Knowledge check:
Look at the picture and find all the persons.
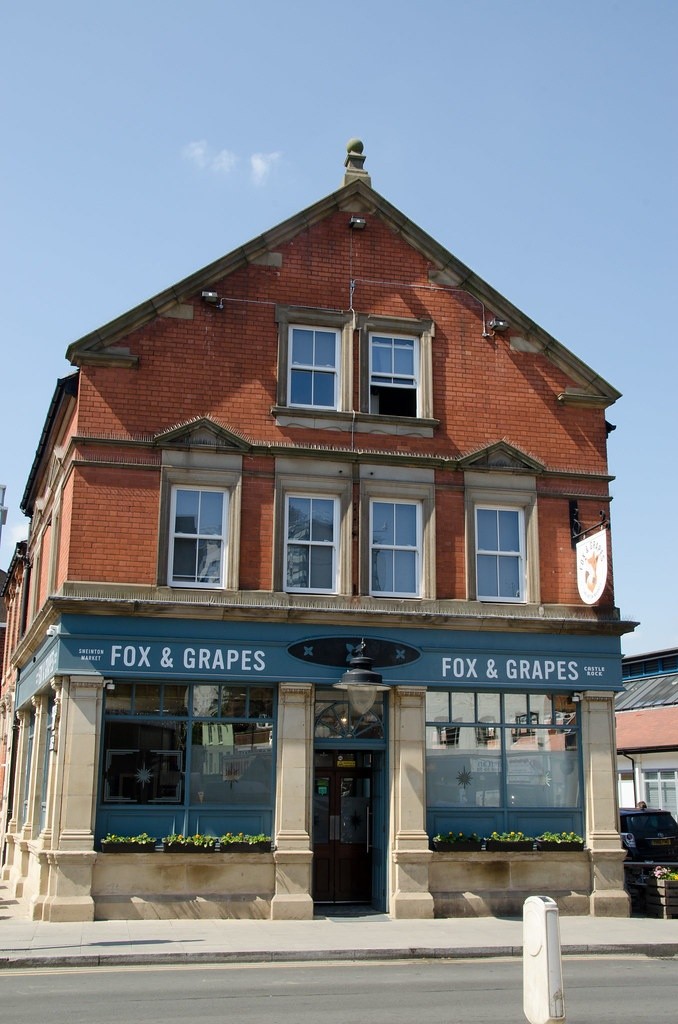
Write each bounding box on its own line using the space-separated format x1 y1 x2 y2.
637 801 647 809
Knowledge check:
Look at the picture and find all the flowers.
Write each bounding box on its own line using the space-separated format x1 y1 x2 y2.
220 832 272 846
162 833 215 847
649 866 677 880
434 831 480 844
100 833 158 844
541 831 584 844
487 831 534 842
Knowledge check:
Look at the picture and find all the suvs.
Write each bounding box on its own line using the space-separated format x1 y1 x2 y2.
618 801 678 896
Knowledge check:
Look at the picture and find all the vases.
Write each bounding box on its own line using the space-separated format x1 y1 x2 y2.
217 838 273 852
97 842 156 852
163 842 217 853
645 877 678 919
486 839 534 850
535 837 585 851
429 838 482 851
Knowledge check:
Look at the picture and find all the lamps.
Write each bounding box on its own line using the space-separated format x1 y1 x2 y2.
489 319 510 332
350 214 367 231
331 641 391 716
200 290 219 304
